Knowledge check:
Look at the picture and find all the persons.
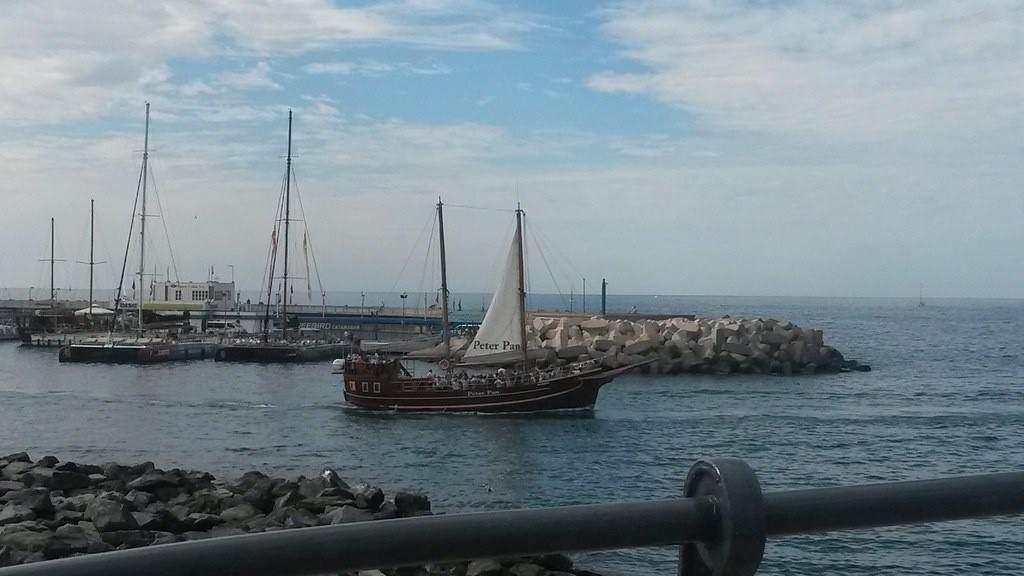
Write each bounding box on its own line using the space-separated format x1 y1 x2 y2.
231 335 341 347
352 349 556 391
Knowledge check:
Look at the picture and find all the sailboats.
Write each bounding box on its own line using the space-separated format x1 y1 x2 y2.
332 194 658 417
20 95 472 364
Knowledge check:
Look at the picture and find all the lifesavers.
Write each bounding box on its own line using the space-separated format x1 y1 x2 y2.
439 359 450 371
222 338 229 346
